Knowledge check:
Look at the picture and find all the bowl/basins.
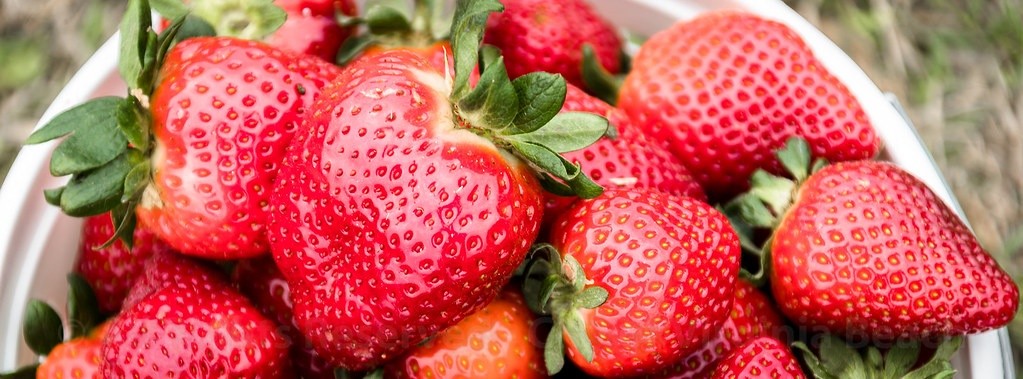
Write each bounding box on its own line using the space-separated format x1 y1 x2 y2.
1 0 1017 379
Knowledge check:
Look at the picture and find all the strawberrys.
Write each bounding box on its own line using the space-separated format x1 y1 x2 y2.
0 0 1021 379
